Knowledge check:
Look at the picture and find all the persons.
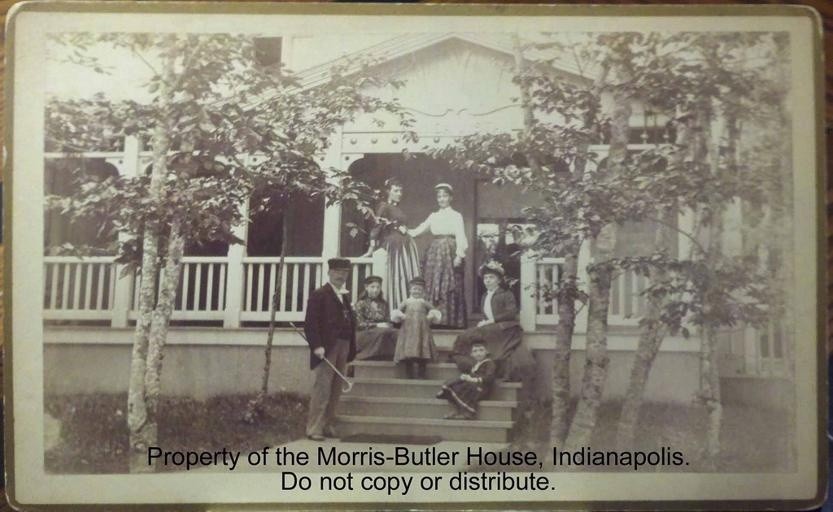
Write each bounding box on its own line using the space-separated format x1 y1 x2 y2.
304 257 356 441
362 178 421 319
353 276 393 363
390 277 443 378
436 339 497 419
451 260 524 381
408 182 469 327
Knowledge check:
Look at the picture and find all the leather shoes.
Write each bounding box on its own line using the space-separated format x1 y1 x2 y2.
309 434 326 440
322 425 340 438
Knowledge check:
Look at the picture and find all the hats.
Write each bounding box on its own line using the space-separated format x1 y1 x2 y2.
365 277 382 282
406 277 426 290
327 259 353 272
470 337 488 348
477 261 506 284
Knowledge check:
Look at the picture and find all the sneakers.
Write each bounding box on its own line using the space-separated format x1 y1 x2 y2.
435 387 451 399
443 407 471 420
